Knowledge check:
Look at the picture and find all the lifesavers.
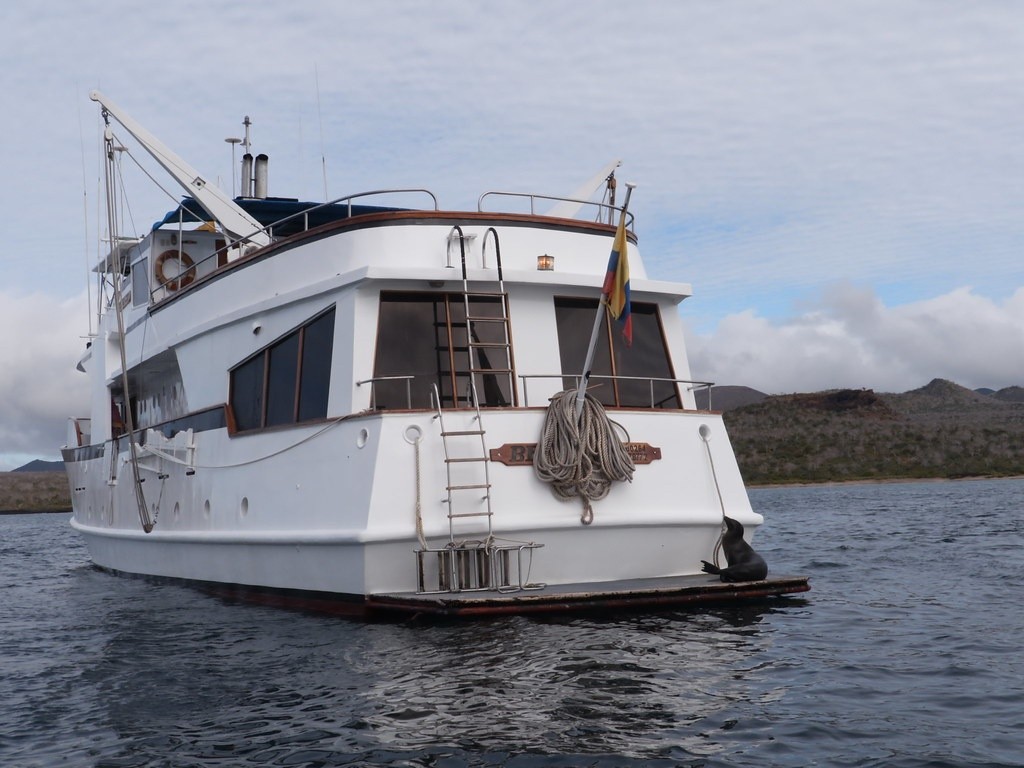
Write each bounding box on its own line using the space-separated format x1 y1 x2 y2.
155 248 196 290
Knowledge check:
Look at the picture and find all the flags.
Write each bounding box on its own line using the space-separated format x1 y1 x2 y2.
600 207 633 351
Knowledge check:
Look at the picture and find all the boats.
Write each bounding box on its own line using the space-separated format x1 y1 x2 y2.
59 91 814 623
